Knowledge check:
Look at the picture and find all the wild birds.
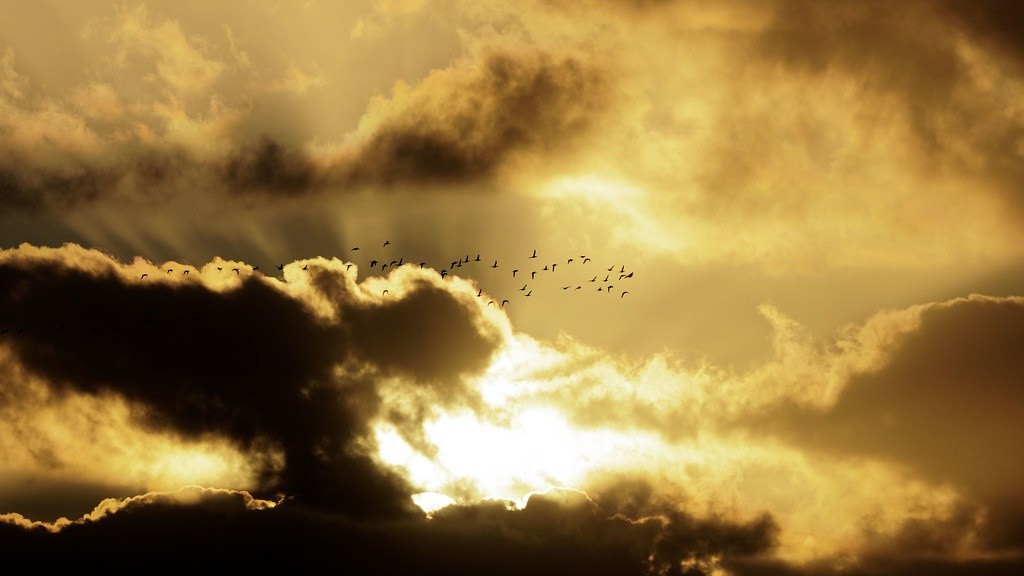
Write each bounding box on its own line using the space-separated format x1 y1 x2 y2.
138 238 637 308
